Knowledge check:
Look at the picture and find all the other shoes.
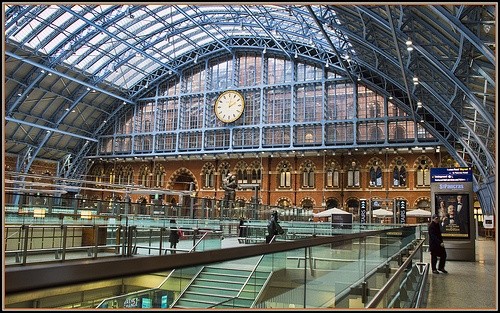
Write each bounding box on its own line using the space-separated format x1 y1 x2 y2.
438 269 448 273
432 270 439 274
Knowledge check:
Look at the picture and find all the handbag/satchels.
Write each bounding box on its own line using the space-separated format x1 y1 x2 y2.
177 230 182 237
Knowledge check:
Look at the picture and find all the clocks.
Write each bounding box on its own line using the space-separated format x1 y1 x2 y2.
213 89 246 123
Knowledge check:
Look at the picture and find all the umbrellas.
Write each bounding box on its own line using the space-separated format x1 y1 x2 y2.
281 209 314 216
314 208 349 218
405 209 431 223
366 209 394 223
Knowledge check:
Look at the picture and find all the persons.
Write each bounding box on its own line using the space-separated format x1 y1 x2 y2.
169 218 179 253
222 173 237 216
309 216 313 222
238 217 244 237
438 195 468 234
428 215 447 274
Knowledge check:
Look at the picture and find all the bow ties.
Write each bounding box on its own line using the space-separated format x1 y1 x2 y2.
458 203 461 205
450 217 454 219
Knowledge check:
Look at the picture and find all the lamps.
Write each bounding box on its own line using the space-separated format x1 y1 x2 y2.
324 58 329 68
193 53 199 64
417 98 422 109
262 44 267 54
294 49 298 58
168 63 174 75
144 77 150 89
405 35 414 53
357 73 362 82
412 72 420 85
389 92 394 100
